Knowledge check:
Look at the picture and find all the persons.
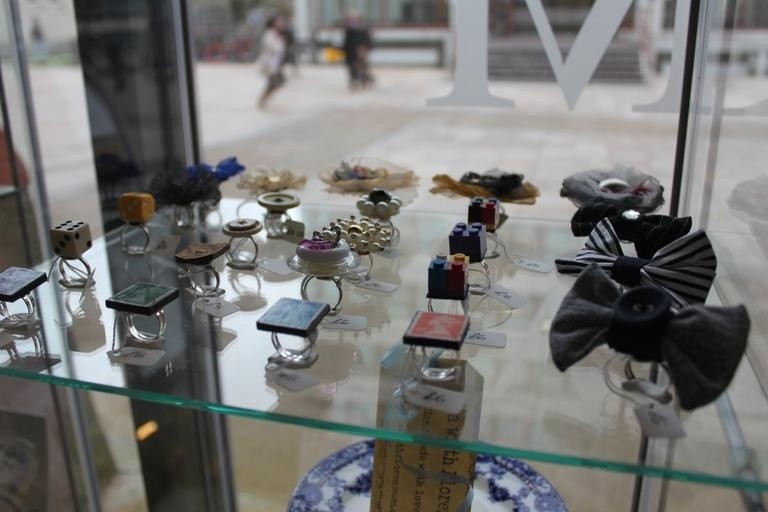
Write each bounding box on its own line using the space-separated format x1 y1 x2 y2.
341 9 375 94
254 12 296 108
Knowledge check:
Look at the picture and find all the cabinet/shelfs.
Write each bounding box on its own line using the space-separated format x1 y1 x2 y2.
0 0 767 512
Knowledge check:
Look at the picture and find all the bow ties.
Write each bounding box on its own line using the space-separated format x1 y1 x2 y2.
548 261 752 413
555 217 718 310
571 203 693 260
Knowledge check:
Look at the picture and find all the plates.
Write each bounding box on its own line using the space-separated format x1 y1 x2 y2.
285 438 567 512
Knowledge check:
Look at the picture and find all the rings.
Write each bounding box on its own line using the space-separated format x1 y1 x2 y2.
1 189 509 413
